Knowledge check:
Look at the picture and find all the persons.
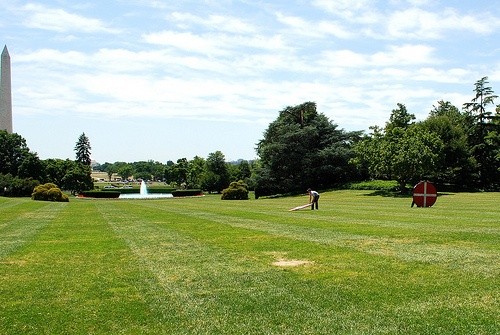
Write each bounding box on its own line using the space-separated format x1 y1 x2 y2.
307 188 320 211
409 187 421 208
